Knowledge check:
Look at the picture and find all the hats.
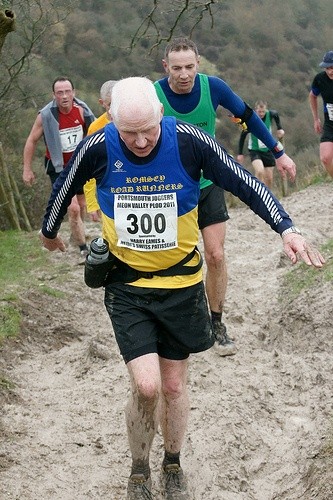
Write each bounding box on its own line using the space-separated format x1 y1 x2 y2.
318 51 333 68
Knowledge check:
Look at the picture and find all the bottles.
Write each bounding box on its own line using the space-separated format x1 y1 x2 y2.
86 237 109 264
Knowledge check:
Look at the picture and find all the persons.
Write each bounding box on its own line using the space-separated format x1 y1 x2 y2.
236 99 285 191
152 38 295 356
308 51 333 181
23 77 121 256
42 76 326 500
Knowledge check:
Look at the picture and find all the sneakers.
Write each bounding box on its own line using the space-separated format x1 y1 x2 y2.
126 471 152 500
211 321 237 356
78 247 88 265
161 463 184 500
